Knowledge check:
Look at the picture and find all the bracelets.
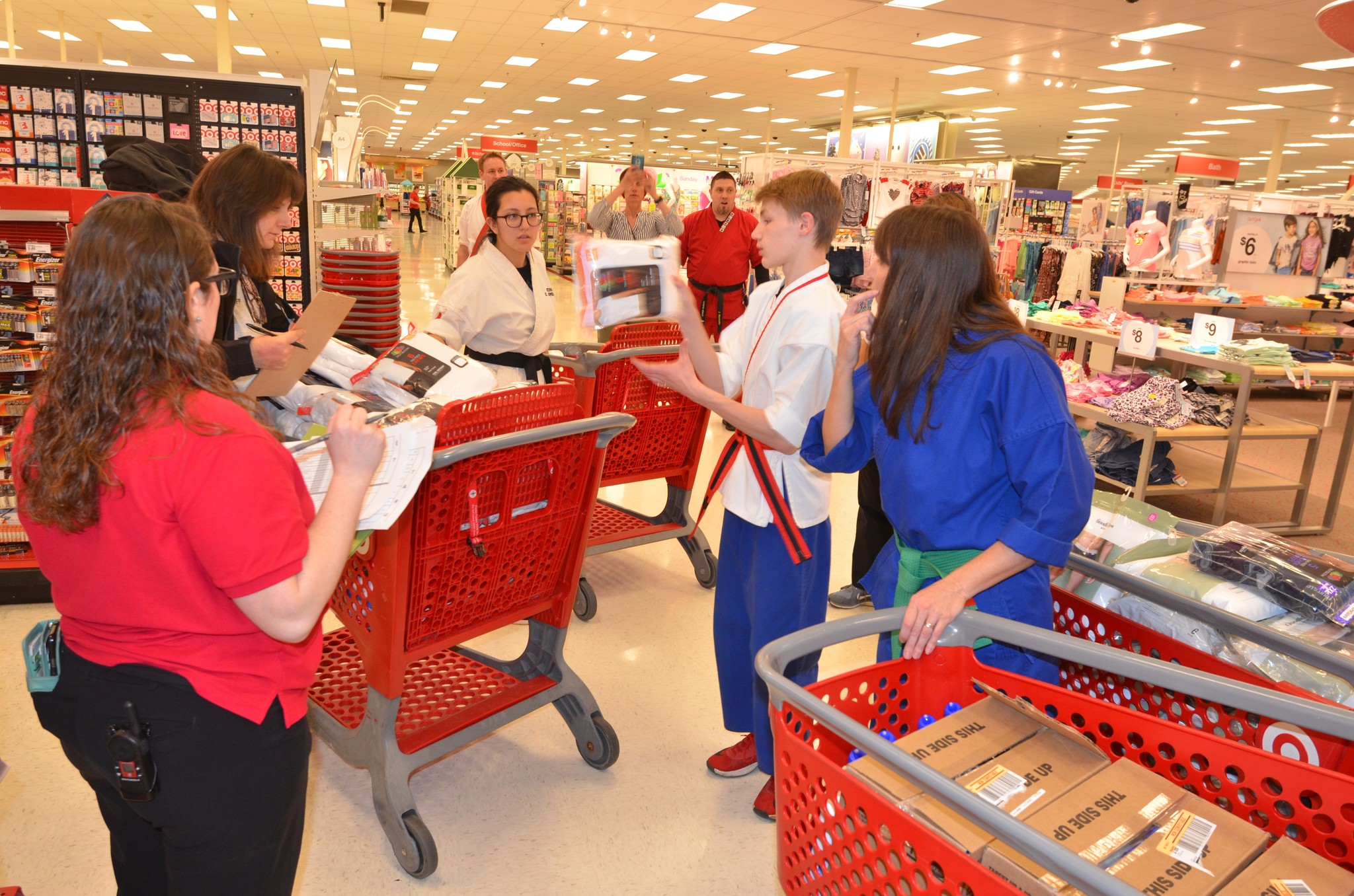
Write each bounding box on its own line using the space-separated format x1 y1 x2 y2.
654 197 663 204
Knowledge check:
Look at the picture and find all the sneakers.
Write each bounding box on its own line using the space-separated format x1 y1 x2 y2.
706 733 759 778
828 585 873 609
753 775 776 821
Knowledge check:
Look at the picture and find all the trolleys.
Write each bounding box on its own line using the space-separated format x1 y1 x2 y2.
752 599 1354 896
1046 517 1354 878
539 318 719 624
297 378 640 880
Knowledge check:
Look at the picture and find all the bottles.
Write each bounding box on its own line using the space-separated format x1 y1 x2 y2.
372 237 378 251
363 237 369 251
354 237 360 251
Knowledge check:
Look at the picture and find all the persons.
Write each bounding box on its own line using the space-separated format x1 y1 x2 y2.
188 144 381 381
423 193 429 211
457 151 541 268
408 185 428 233
663 178 680 216
557 179 564 191
800 205 1096 718
1275 215 1324 276
1170 218 1213 279
631 170 848 822
1123 210 1170 271
12 193 387 896
698 179 712 211
676 170 770 343
828 192 976 609
1088 203 1102 234
423 176 556 384
587 165 684 241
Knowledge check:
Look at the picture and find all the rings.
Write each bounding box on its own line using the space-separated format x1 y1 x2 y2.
924 622 935 628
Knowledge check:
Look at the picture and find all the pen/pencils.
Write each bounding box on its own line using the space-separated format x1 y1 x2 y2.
285 413 390 452
245 322 310 349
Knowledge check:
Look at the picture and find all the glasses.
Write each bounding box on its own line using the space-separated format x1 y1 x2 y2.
498 213 543 227
205 267 237 298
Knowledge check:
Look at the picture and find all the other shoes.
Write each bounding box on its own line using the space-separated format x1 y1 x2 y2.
408 230 415 233
420 229 427 233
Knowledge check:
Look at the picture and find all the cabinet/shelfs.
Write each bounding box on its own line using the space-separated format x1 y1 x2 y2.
1026 278 1354 537
317 176 581 273
